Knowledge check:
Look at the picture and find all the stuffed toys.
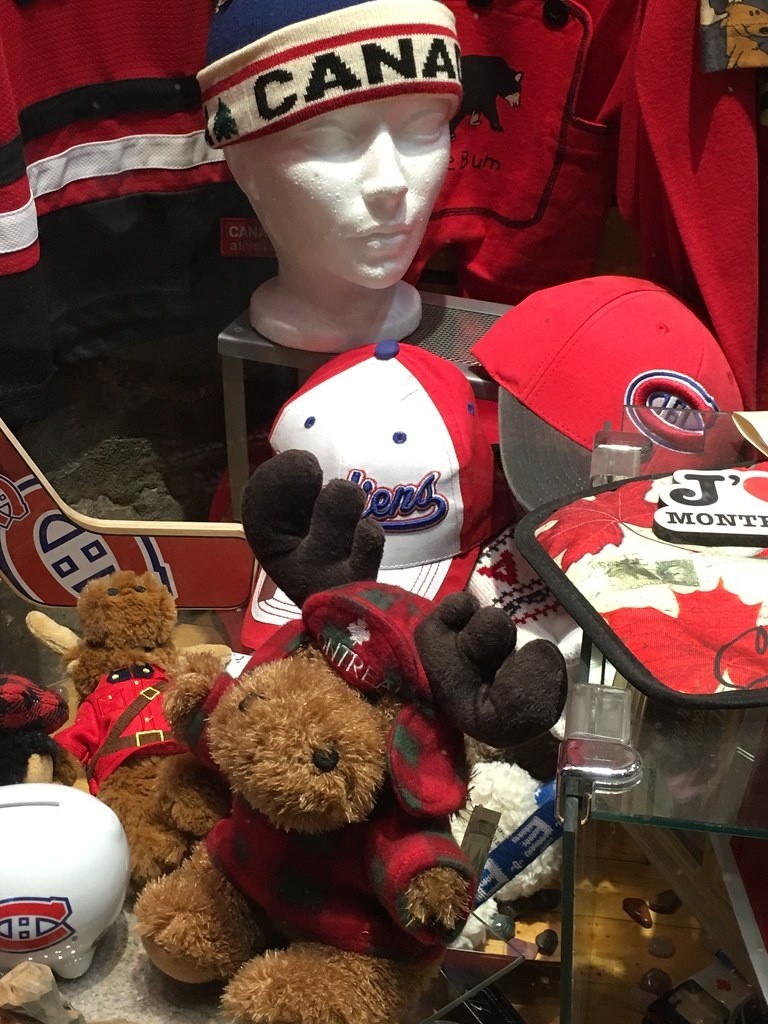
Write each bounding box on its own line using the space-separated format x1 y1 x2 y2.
26 568 222 885
134 450 567 1024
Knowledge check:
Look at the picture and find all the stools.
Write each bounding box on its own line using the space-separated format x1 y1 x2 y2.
215 290 514 521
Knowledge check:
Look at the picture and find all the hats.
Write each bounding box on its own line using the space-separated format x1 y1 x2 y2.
193 1 472 151
235 339 497 656
468 272 748 517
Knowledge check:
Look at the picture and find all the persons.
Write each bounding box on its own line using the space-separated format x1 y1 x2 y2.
201 1 465 354
